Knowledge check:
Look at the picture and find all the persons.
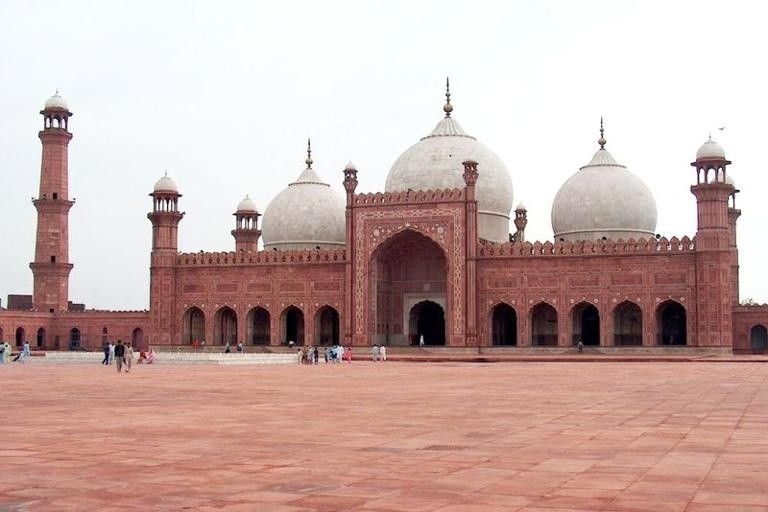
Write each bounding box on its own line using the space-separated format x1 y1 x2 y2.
288 338 295 348
379 345 387 361
0 340 31 366
225 340 231 352
371 344 380 364
419 333 424 348
297 343 353 365
101 340 158 372
578 340 583 352
235 340 246 353
